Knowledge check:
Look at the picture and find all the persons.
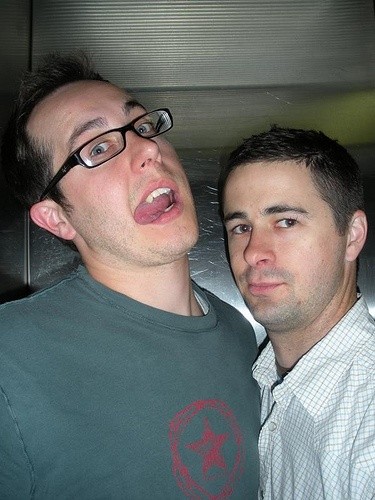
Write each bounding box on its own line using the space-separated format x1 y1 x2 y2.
0 49 267 499
213 123 375 500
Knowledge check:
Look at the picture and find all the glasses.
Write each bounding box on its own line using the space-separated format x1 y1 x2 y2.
38 108 173 203
